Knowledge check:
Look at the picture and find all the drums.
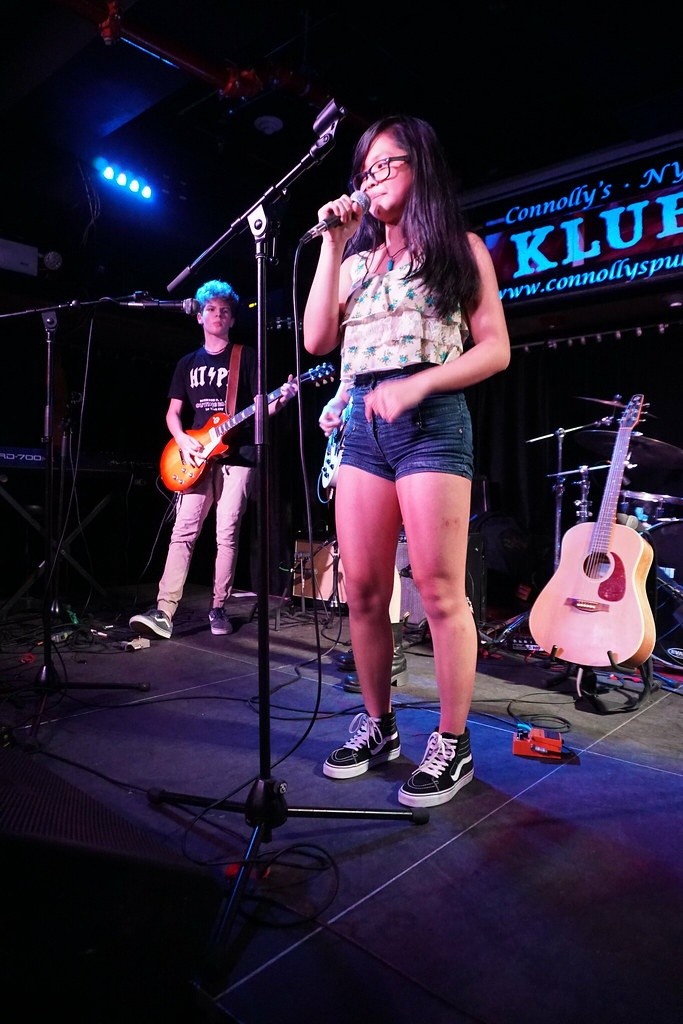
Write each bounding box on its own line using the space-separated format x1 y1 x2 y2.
639 521 683 668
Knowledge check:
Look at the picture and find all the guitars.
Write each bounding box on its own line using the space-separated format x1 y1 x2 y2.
160 363 344 493
529 393 656 671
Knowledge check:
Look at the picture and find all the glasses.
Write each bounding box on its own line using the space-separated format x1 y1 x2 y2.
353 156 410 192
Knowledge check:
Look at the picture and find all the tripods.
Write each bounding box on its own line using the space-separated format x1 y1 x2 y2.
147 98 430 978
295 533 350 628
0 289 150 749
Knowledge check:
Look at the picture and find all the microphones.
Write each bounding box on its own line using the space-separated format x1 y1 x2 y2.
120 297 200 315
300 191 370 245
291 568 318 576
399 570 413 579
607 461 630 486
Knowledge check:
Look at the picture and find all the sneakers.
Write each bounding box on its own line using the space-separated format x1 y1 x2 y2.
209 607 233 635
323 707 400 778
129 609 174 638
398 727 474 807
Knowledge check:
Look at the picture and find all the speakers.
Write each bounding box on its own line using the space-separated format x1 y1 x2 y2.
291 532 350 614
387 533 485 631
0 745 230 1024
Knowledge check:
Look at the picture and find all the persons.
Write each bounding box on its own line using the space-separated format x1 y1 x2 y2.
315 287 409 695
127 278 304 639
300 117 512 807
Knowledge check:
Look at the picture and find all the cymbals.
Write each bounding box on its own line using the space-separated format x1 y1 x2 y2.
579 397 656 420
575 430 681 464
